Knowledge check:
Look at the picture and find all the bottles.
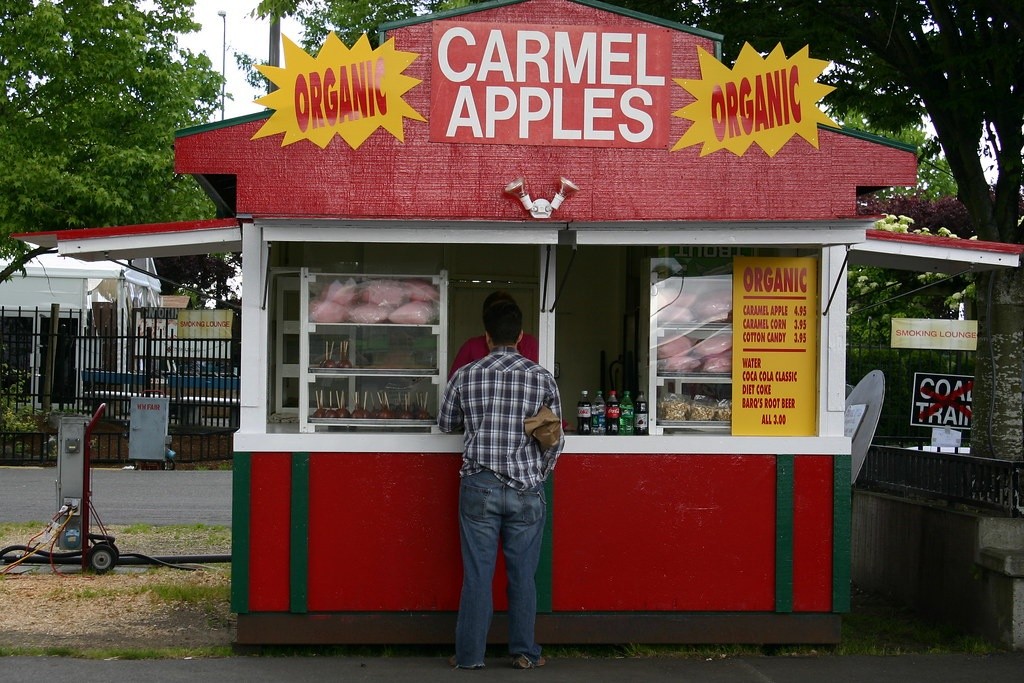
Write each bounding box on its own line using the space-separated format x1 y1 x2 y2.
619 390 634 434
592 390 606 434
606 390 619 433
576 390 592 434
634 392 647 434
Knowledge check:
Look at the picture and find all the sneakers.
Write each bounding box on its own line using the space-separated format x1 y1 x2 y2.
513 656 546 669
449 655 478 669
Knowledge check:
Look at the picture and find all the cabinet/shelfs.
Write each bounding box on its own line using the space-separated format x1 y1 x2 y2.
297 265 448 434
639 257 731 436
273 276 357 419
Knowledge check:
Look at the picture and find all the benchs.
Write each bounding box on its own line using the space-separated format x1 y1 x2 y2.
971 515 1023 651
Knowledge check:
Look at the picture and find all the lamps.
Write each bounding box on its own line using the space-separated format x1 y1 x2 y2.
505 176 581 219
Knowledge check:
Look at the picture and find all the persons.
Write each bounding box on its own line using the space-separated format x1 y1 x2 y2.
447 290 577 433
435 299 562 670
